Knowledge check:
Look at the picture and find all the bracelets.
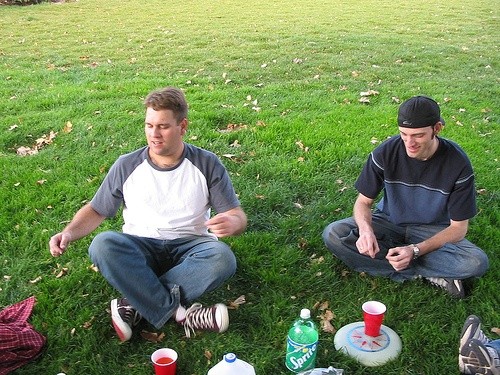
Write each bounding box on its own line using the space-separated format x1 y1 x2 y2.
410 243 420 260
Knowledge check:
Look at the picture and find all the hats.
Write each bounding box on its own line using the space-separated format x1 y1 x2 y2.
397 96 445 128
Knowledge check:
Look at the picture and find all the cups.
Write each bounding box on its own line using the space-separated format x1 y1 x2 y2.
362 301 387 337
151 347 178 375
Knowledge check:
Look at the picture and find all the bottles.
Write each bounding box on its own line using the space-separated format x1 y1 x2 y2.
207 352 256 375
285 308 319 375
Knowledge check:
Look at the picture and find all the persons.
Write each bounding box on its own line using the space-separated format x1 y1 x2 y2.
322 96 489 299
49 86 247 344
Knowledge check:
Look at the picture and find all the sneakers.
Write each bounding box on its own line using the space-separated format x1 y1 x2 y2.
176 302 229 339
110 298 142 342
458 315 500 375
413 273 464 300
